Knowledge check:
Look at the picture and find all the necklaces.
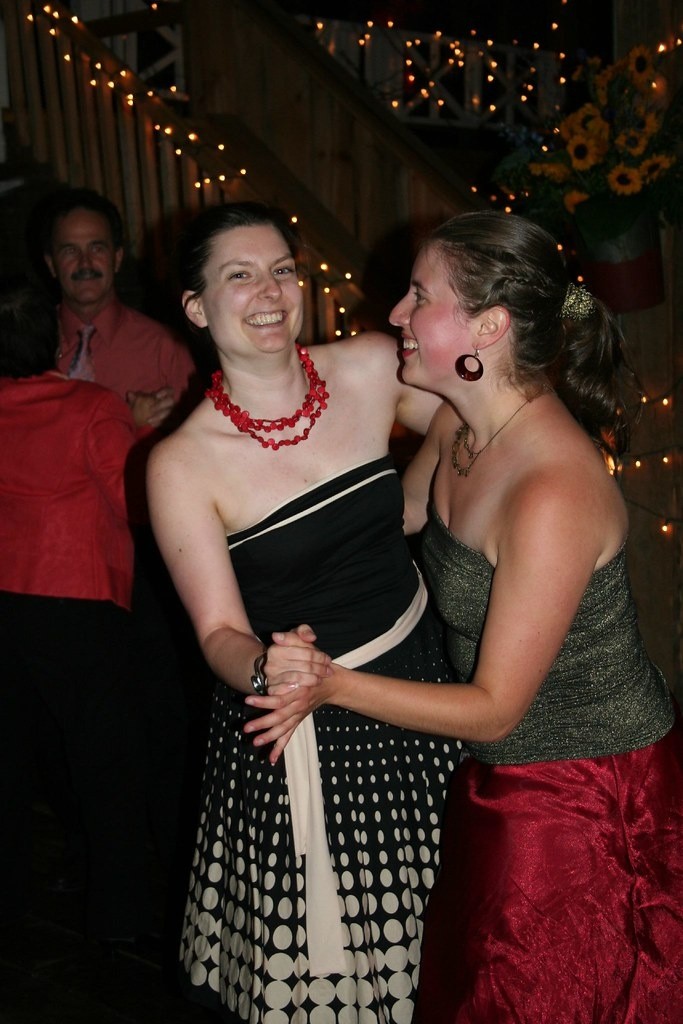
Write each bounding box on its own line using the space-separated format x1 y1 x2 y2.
204 344 329 449
450 398 529 477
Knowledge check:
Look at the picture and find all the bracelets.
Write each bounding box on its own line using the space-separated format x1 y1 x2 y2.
250 655 269 694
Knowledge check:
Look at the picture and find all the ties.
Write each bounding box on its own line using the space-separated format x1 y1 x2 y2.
66 325 97 382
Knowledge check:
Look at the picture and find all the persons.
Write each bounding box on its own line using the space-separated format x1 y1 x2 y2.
0 194 205 943
147 198 466 1024
244 208 682 1024
